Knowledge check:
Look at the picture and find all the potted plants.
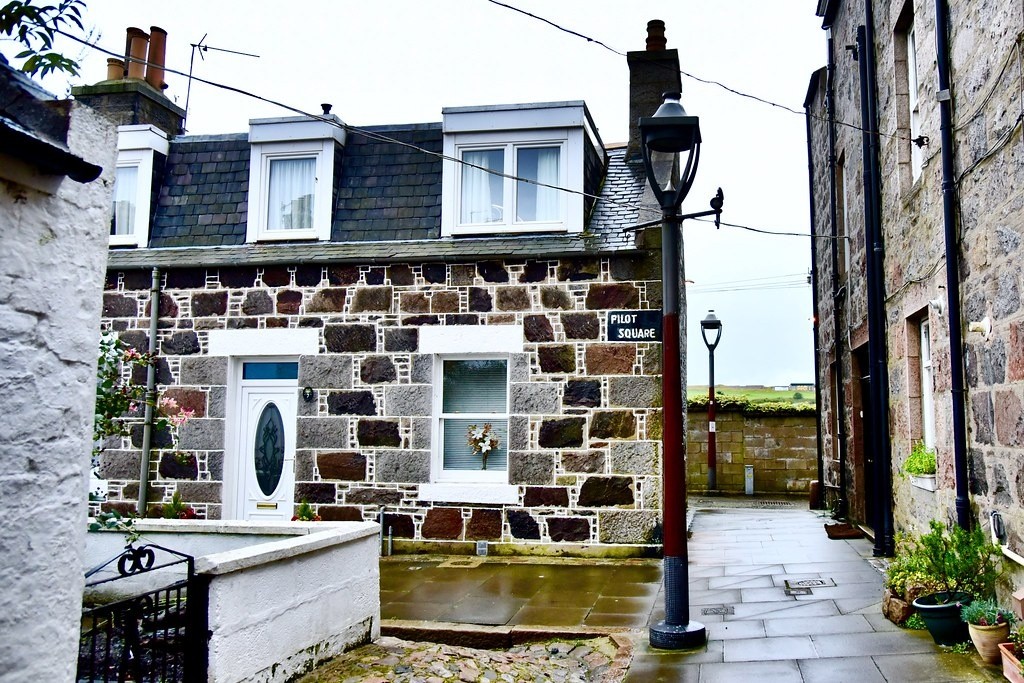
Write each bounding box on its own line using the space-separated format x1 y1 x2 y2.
910 517 1016 646
902 442 936 492
998 627 1024 683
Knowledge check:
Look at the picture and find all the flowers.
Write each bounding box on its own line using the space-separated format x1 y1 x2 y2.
164 490 197 518
955 598 1017 628
290 499 322 521
90 329 196 456
464 422 503 463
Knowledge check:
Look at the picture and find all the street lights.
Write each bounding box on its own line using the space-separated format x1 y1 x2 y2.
700 309 723 490
639 87 705 649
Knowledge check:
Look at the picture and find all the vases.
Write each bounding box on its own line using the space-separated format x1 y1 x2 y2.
967 621 1010 663
481 452 488 470
